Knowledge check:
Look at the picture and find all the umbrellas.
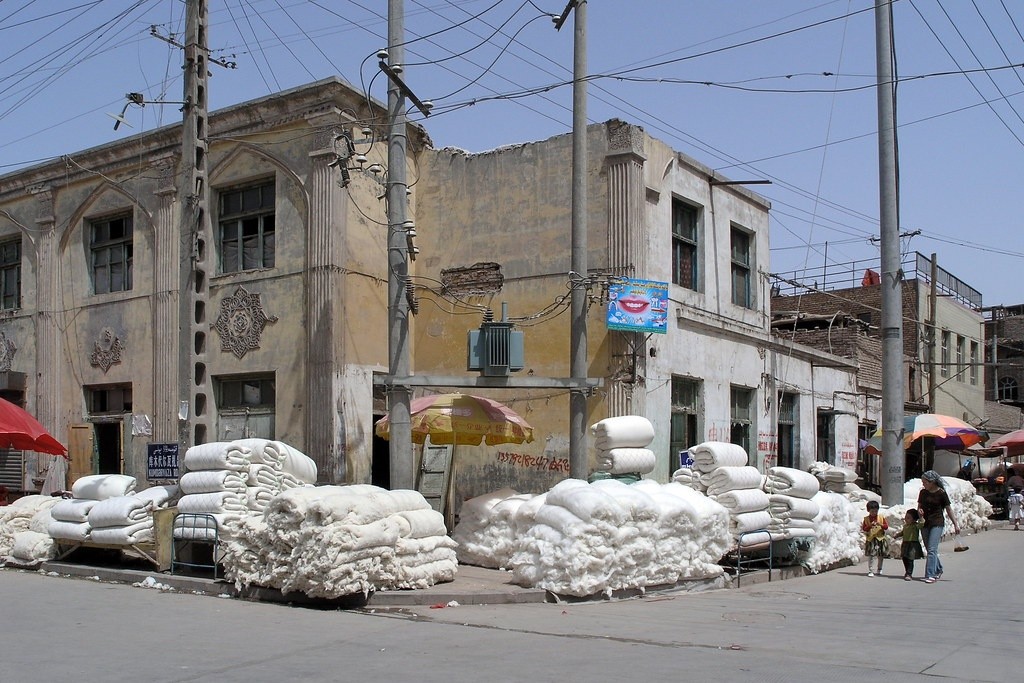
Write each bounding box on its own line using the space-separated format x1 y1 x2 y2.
989 429 1024 458
375 392 535 533
864 412 991 472
0 397 68 459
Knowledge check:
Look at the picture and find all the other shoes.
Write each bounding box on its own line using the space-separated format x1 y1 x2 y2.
904 574 912 581
876 567 882 575
1013 524 1020 531
868 570 875 577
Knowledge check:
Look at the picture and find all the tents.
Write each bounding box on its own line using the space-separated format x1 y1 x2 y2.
947 443 1008 492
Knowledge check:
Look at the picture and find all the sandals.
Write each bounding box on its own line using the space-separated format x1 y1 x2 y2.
925 573 942 583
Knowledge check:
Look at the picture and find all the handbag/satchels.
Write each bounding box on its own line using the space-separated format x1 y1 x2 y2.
954 533 969 552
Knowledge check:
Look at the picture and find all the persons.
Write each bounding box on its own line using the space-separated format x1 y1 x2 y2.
895 509 925 581
862 501 888 577
957 461 975 481
917 470 961 583
1004 467 1024 530
